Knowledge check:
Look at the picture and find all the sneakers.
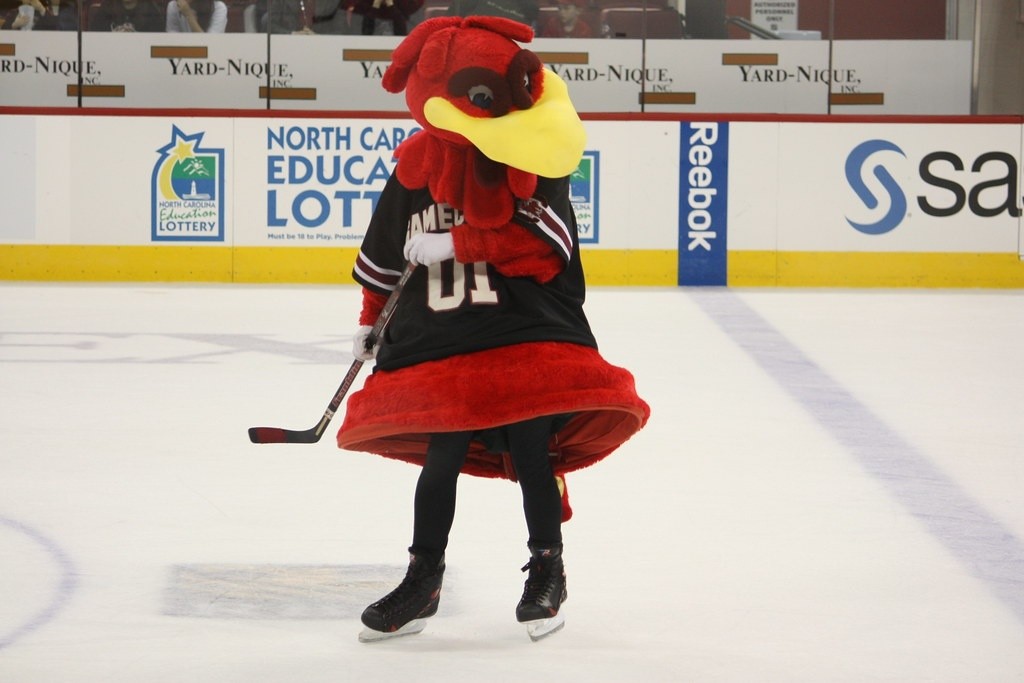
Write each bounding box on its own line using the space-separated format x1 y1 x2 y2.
517 542 568 641
359 546 446 640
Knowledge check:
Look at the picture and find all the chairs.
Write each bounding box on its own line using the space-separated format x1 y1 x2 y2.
536 0 687 40
225 0 313 33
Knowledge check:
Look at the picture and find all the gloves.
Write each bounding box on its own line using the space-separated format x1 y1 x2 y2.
352 325 380 361
403 233 455 267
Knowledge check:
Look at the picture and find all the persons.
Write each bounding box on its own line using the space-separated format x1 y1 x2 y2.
352 14 587 636
0 0 596 38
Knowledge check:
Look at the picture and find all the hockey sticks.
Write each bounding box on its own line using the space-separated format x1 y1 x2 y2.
248 259 417 444
